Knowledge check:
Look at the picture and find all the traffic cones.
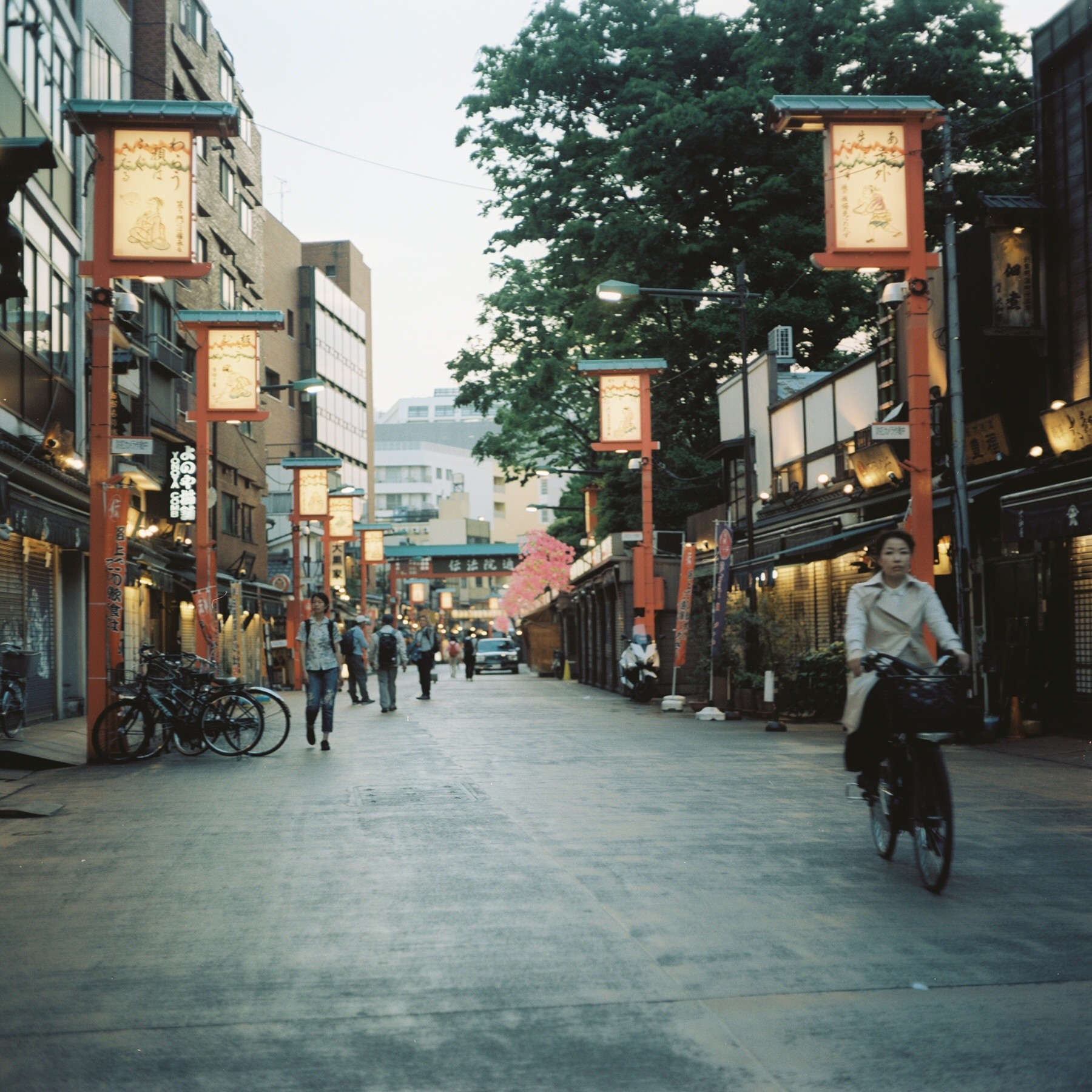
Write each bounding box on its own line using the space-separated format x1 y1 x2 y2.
1002 695 1027 742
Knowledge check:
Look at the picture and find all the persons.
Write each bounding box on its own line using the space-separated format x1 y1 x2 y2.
447 630 475 682
838 530 972 828
342 614 440 713
296 592 344 750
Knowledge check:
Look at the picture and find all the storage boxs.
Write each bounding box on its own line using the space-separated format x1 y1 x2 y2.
2 651 42 677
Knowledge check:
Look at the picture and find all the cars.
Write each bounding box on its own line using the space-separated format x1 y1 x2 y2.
473 637 523 675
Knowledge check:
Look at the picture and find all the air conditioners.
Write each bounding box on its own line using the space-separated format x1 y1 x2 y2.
767 325 793 358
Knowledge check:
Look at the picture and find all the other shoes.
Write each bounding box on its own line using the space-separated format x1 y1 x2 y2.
381 708 387 713
389 706 396 710
416 695 430 699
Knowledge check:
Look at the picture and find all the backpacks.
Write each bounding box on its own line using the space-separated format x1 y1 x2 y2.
340 630 360 654
449 643 457 657
463 638 473 655
426 626 440 653
375 628 398 666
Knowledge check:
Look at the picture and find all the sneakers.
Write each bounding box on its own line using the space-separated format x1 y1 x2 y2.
361 698 375 705
321 740 330 750
307 727 316 745
352 700 361 704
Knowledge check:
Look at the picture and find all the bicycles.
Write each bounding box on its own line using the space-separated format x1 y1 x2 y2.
547 645 563 680
843 647 983 895
1 642 42 742
92 643 292 767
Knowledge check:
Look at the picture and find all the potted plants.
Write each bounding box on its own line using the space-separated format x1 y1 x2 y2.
698 637 778 712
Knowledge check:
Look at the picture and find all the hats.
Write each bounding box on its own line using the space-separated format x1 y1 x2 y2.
355 615 370 623
379 613 393 624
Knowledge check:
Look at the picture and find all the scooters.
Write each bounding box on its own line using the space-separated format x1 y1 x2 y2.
619 623 661 704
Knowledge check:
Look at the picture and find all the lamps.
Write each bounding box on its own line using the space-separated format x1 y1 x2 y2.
46 438 60 449
886 471 898 482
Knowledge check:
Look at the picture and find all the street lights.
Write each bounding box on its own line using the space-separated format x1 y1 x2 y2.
594 259 762 676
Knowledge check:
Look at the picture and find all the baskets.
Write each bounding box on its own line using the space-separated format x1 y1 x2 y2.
884 673 972 735
